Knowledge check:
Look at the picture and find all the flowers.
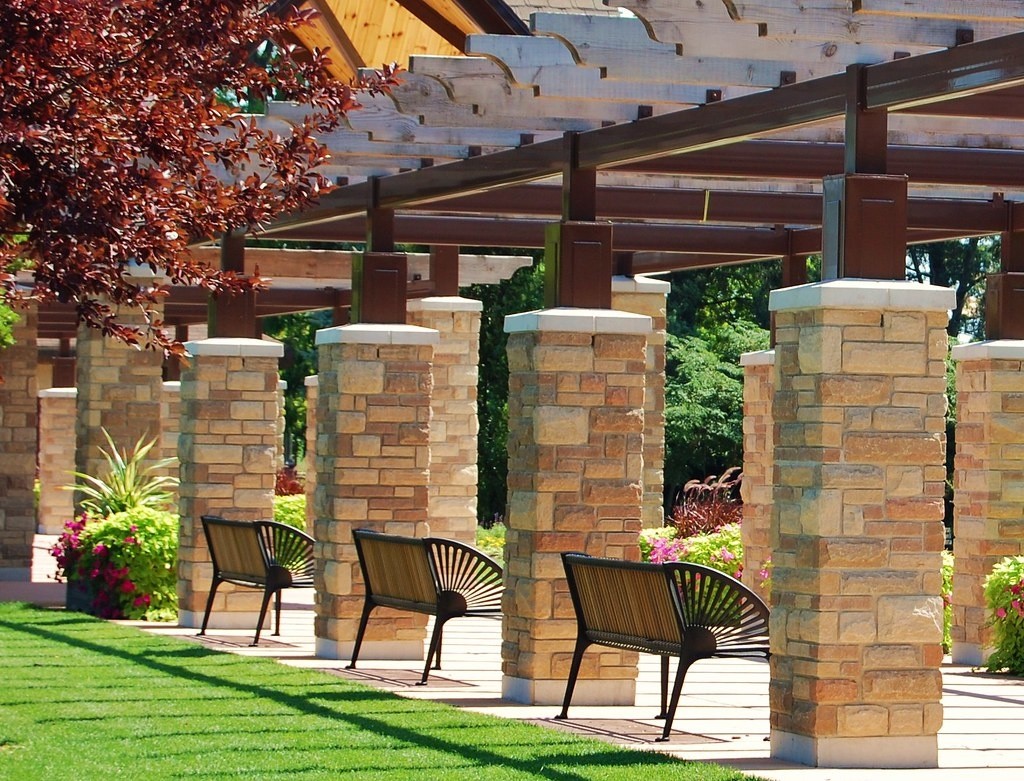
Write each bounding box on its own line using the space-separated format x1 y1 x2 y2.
44 419 179 621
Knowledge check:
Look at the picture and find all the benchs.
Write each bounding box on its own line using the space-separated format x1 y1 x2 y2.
196 515 316 648
345 529 504 686
553 550 772 746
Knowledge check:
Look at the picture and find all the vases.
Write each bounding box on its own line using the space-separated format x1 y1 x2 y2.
65 575 94 612
